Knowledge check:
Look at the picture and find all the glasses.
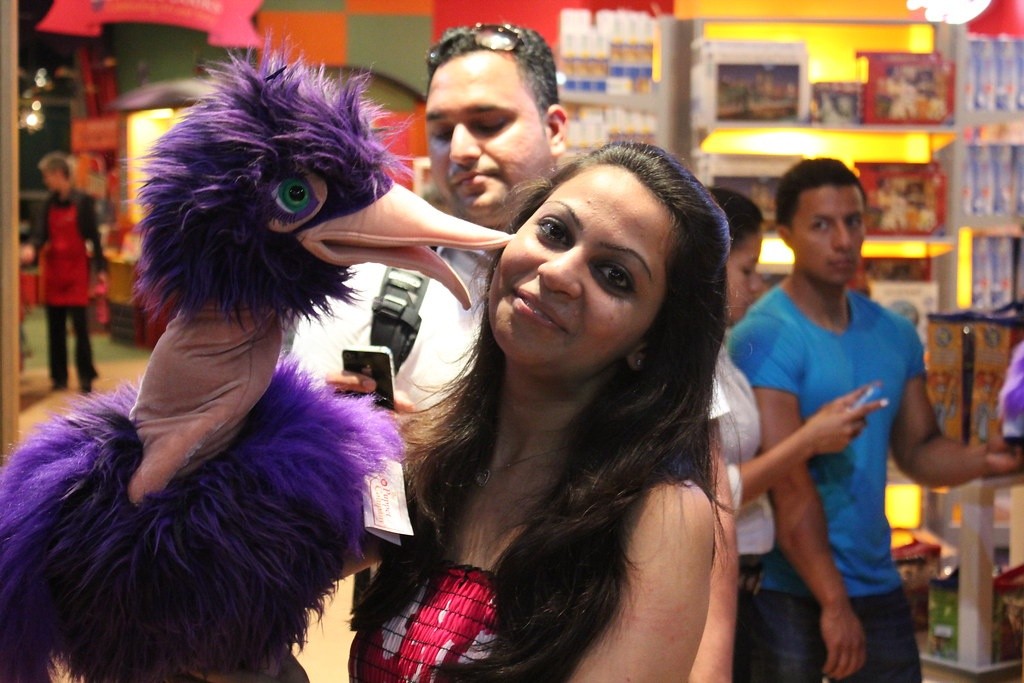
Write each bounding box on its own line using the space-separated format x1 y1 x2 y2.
425 23 554 102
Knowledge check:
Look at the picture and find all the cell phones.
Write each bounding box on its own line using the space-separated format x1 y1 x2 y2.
342 345 394 411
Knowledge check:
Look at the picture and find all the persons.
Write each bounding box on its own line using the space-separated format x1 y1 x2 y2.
701 157 1024 683
290 22 740 683
20 152 110 396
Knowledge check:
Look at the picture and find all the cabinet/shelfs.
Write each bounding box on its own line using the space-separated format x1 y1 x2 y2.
548 82 660 181
957 106 1024 326
918 475 1024 683
661 17 962 322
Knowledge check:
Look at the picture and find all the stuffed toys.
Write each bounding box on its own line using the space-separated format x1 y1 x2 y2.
0 31 514 683
